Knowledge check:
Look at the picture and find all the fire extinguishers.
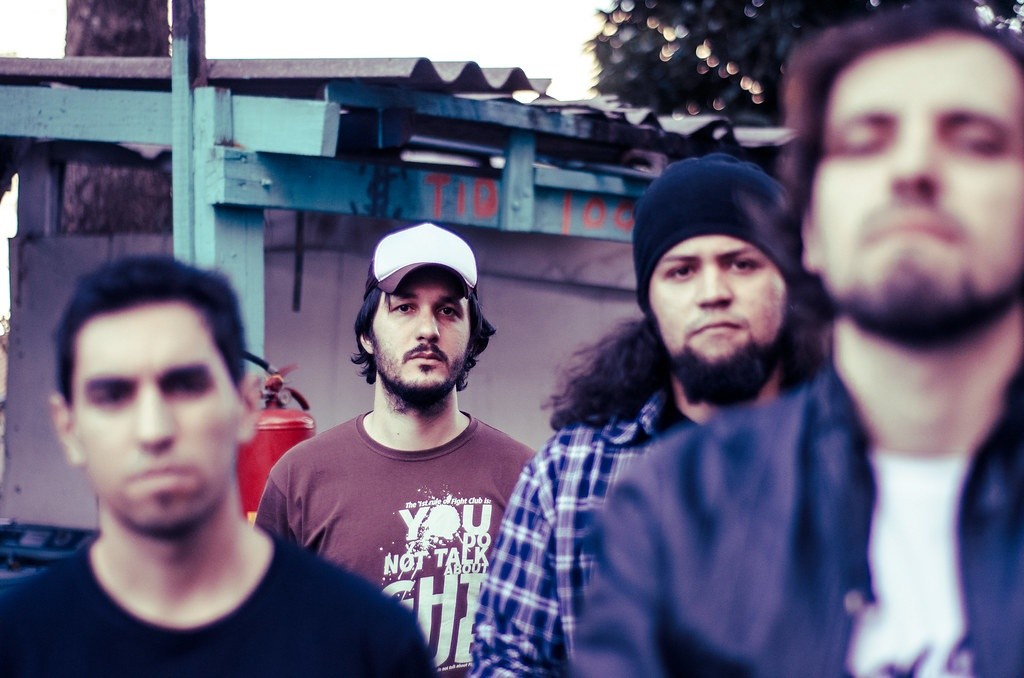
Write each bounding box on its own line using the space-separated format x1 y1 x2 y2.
237 352 317 532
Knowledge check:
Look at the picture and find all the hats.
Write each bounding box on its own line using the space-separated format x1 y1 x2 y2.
362 222 478 301
631 153 799 316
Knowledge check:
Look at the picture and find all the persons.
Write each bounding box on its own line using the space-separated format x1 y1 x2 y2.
255 222 536 678
0 252 436 678
469 153 827 678
579 14 1024 678
